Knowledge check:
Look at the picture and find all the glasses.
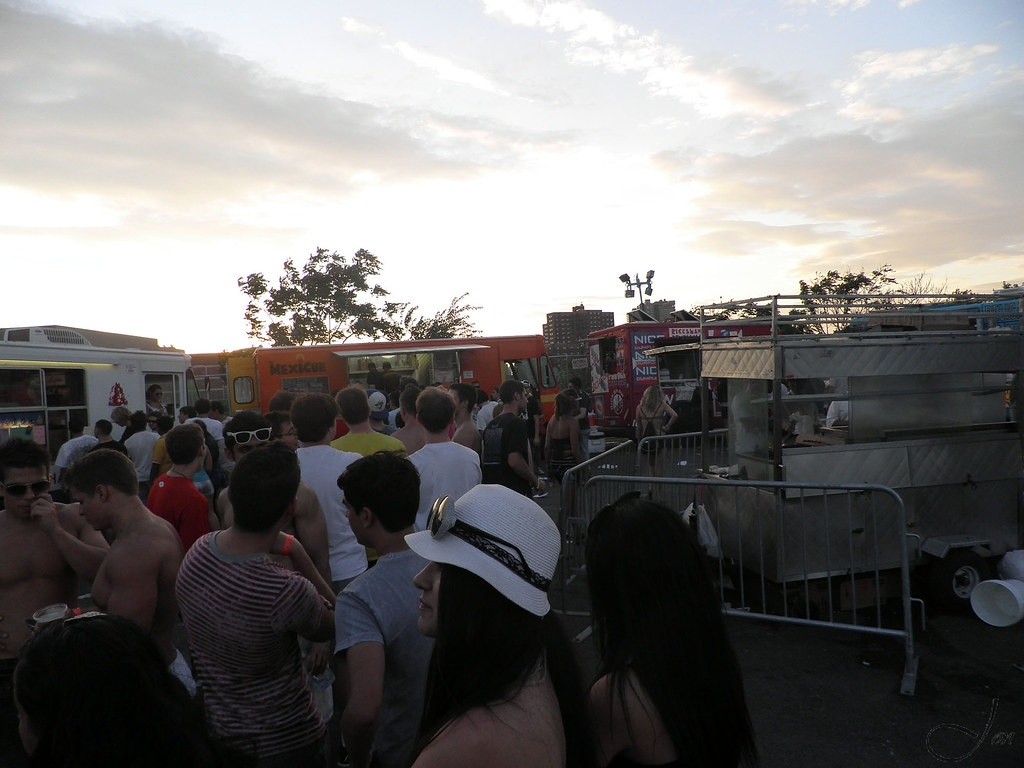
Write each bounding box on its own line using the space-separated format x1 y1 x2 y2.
0 476 51 496
225 426 273 445
147 420 157 424
154 391 163 396
587 490 641 535
279 426 295 436
424 496 533 577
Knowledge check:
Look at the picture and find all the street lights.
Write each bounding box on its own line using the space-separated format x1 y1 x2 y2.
619 269 655 312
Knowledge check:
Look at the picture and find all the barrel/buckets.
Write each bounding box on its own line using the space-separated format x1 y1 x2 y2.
970 578 1024 626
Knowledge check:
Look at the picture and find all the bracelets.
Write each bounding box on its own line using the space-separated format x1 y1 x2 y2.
283 535 294 555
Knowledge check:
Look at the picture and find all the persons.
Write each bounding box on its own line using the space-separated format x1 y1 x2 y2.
0 361 849 768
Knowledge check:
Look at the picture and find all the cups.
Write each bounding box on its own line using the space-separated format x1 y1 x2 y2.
33 603 68 623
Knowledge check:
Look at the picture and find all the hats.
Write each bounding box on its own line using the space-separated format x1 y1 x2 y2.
365 389 390 419
404 483 561 618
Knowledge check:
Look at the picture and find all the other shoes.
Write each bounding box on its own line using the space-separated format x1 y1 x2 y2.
335 714 350 767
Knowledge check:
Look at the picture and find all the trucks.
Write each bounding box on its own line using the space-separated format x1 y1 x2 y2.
226 334 561 446
587 321 783 434
0 326 214 501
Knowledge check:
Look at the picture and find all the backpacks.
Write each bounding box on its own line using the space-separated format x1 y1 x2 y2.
640 402 663 453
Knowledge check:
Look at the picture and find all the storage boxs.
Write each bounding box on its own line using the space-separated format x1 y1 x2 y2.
47 387 74 404
796 574 890 613
46 371 71 387
